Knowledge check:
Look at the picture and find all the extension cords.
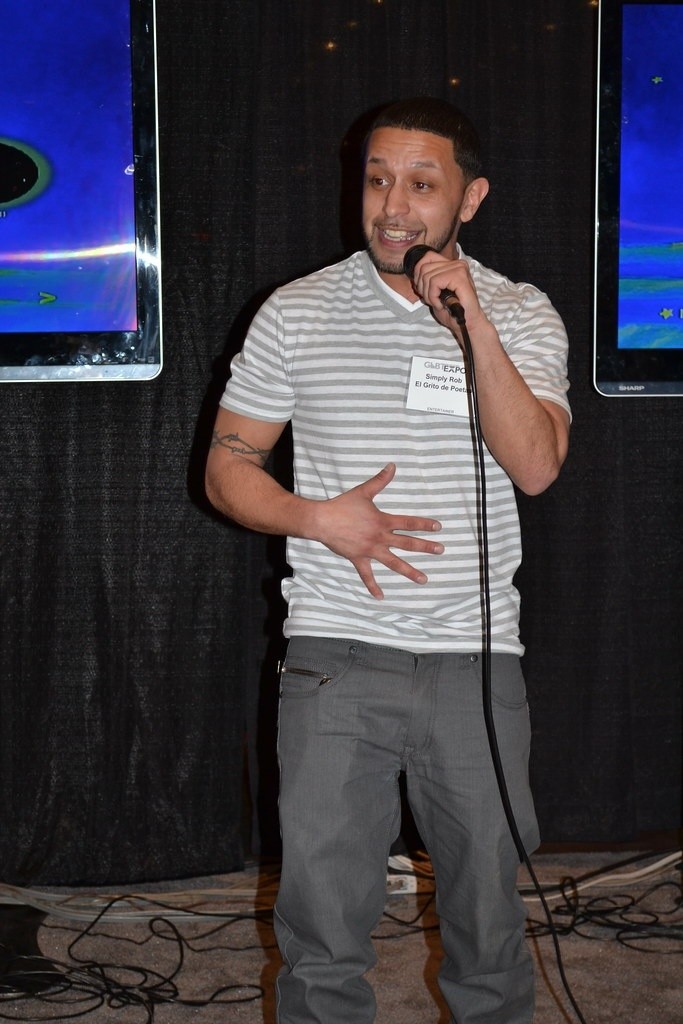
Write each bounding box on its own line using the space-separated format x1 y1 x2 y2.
386 873 436 895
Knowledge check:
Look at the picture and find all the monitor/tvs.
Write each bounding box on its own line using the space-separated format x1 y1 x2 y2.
0 0 164 384
594 0 683 398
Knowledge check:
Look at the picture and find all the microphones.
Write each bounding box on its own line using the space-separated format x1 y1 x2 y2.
402 244 464 317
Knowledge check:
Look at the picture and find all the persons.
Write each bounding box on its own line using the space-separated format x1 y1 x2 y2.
204 96 573 1024
